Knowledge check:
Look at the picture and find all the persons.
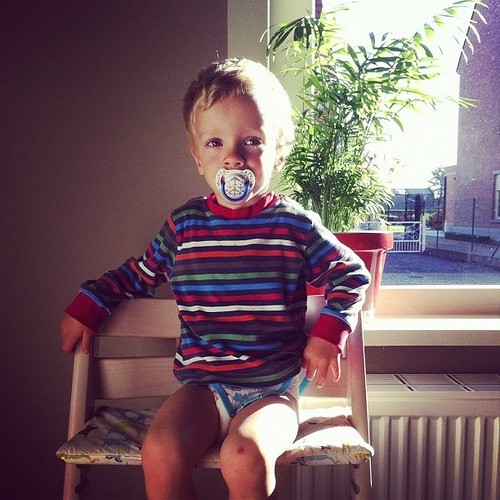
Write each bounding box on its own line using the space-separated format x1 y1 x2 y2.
61 59 372 500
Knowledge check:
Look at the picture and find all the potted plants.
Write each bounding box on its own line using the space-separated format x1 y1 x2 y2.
256 0 500 327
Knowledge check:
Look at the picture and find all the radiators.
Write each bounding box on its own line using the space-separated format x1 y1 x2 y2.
294 371 500 500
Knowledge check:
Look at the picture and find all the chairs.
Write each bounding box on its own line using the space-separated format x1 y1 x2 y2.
56 290 374 500
